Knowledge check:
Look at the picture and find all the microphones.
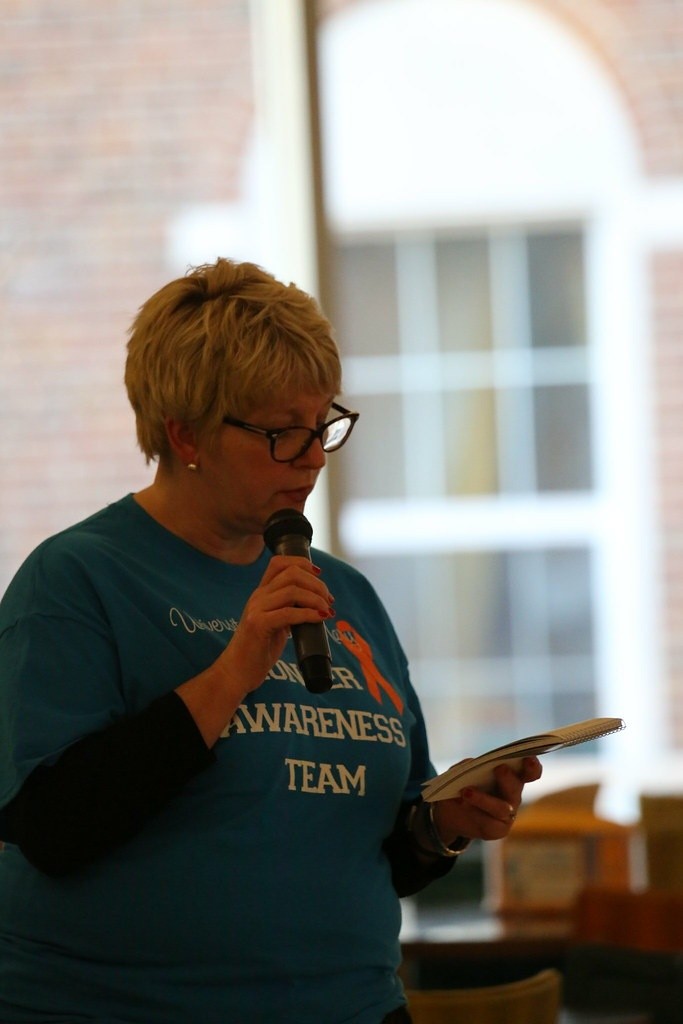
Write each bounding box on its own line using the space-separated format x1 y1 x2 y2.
262 508 333 695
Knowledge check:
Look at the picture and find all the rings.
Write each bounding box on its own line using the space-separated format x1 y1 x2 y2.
504 803 516 824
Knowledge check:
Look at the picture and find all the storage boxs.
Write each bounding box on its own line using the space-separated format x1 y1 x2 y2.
483 784 683 922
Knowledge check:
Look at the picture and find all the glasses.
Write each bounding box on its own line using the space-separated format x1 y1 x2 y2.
222 403 360 463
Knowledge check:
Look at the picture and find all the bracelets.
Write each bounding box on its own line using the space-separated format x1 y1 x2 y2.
421 803 470 858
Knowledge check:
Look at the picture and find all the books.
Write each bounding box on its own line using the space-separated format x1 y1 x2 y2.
420 717 622 801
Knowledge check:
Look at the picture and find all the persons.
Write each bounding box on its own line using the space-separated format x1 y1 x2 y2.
0 259 542 1023
